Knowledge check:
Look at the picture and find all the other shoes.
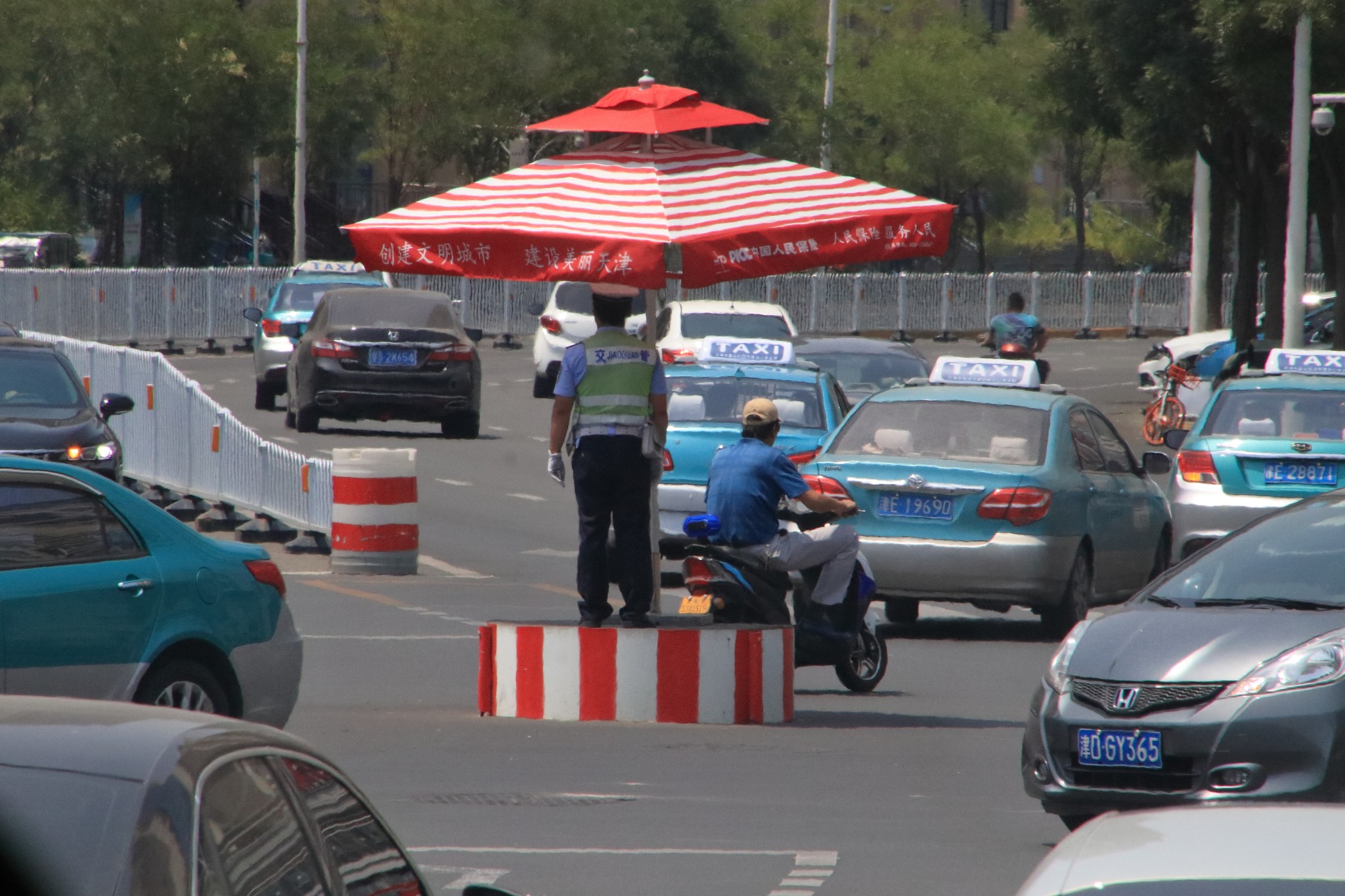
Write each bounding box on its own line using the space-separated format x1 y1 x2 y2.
578 618 603 628
621 614 659 628
798 615 852 641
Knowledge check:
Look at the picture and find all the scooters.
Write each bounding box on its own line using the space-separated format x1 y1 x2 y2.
976 337 1044 361
676 498 888 693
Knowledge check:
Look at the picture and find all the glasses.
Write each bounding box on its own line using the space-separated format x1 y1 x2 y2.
775 424 780 432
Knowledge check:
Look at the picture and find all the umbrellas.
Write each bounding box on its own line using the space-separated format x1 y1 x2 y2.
332 76 956 614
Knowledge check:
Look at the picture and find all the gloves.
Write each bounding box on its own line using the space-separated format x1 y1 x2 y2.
650 459 663 482
547 450 566 487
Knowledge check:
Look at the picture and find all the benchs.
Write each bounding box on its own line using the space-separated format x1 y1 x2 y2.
1212 419 1345 443
862 430 1037 466
667 393 805 426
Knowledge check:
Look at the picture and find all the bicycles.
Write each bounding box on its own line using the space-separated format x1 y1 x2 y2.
1142 341 1204 447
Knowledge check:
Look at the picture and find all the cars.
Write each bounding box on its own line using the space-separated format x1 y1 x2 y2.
279 288 482 439
785 357 1173 644
240 260 399 375
1022 487 1344 896
1135 291 1345 586
526 280 932 584
0 322 433 896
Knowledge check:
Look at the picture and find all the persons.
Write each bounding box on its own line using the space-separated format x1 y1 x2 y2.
982 292 1050 384
704 397 859 633
547 283 668 626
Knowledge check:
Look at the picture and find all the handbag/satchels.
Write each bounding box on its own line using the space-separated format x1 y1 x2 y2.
641 420 668 459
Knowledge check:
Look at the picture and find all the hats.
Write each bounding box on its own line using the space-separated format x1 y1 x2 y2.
588 282 640 302
741 397 782 426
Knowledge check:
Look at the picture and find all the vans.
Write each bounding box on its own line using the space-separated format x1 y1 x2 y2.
0 230 78 273
